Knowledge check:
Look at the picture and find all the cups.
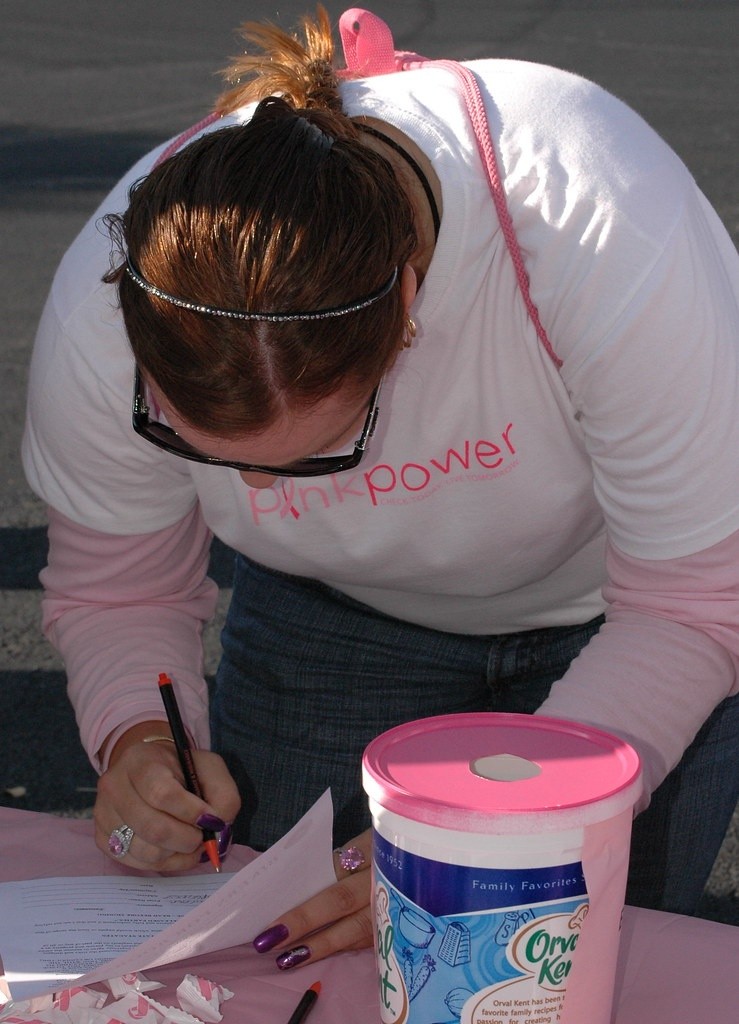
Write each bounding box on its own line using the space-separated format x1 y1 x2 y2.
360 713 642 1024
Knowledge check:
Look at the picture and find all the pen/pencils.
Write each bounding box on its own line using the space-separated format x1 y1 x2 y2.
158 672 221 874
288 981 322 1024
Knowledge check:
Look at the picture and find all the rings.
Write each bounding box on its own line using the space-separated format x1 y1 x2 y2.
106 824 134 859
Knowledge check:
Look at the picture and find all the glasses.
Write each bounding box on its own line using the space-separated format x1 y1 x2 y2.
132 362 386 477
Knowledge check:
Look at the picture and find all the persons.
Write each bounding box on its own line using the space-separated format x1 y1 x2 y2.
20 8 739 972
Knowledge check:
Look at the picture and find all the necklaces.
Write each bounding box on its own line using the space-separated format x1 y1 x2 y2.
351 122 440 245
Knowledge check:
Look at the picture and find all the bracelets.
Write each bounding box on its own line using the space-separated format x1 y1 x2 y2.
142 735 174 743
